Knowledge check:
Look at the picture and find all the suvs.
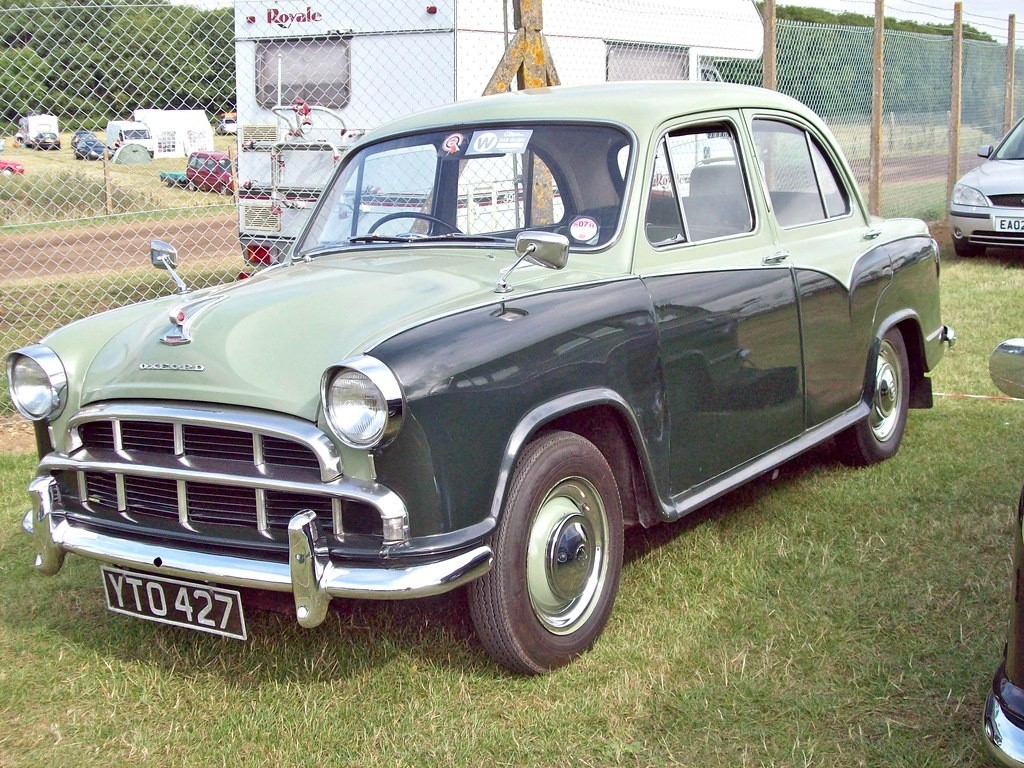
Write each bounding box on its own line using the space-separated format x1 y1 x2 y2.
215 119 237 135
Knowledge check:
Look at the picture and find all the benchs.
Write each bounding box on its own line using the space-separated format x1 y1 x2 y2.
557 191 839 231
555 224 725 246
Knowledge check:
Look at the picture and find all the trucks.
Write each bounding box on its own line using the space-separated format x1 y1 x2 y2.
15 115 59 145
234 0 766 264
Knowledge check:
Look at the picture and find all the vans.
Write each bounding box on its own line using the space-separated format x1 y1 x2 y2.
187 152 237 196
105 121 155 158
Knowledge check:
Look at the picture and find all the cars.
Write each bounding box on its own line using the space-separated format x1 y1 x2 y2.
4 80 956 680
76 139 109 158
71 131 96 149
0 139 4 150
949 115 1024 255
0 160 24 176
32 133 60 150
